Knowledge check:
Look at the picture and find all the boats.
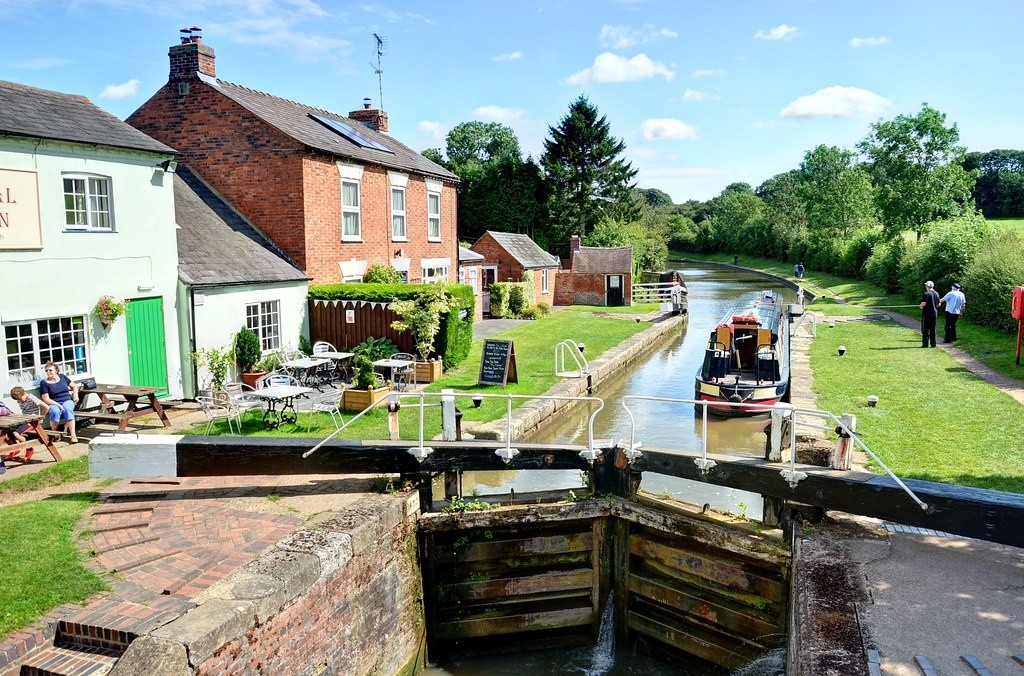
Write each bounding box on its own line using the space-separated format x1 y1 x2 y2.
694 290 790 417
641 269 688 296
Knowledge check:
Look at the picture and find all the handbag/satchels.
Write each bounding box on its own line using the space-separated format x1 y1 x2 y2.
0 401 14 416
78 377 98 392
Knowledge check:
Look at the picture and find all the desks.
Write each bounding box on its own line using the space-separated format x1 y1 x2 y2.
0 413 63 462
246 386 313 430
310 352 356 384
282 358 337 392
68 383 172 432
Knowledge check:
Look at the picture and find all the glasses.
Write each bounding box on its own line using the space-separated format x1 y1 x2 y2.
45 369 56 372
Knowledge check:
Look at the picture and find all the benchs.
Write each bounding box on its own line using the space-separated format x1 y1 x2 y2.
73 412 132 420
26 428 67 439
109 396 184 407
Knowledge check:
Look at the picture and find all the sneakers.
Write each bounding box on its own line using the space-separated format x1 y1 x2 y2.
24 448 35 458
6 450 21 459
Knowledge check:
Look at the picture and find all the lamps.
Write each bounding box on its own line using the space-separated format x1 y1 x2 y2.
394 249 404 257
157 160 179 172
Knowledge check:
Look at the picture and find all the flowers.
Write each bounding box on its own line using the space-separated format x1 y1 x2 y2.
92 295 133 326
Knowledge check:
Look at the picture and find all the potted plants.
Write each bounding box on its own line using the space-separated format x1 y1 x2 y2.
342 360 391 411
236 326 267 391
185 347 237 406
386 271 461 383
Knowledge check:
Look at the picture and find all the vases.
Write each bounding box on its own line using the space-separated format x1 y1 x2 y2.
102 322 108 328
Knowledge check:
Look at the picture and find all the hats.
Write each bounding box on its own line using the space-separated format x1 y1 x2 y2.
952 283 960 289
924 281 934 287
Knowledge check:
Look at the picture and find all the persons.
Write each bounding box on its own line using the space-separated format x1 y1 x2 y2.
794 262 804 279
4 386 49 461
920 281 941 349
38 362 79 444
733 255 739 266
940 283 966 343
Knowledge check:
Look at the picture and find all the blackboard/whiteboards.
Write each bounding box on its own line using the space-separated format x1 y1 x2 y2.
479 339 518 384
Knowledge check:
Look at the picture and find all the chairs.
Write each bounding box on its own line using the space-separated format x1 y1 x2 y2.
195 341 418 436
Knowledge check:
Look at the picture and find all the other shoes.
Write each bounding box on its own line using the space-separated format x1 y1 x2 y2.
930 346 936 349
917 345 928 348
942 339 956 343
71 435 78 443
